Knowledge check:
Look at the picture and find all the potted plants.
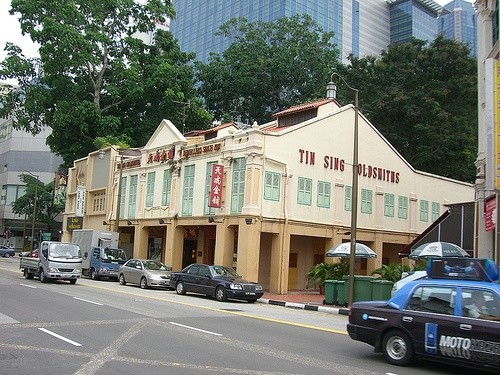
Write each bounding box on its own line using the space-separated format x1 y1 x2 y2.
307 262 334 295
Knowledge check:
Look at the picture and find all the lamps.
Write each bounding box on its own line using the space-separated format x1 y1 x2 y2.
208 217 223 224
103 221 113 225
127 220 139 225
159 219 172 224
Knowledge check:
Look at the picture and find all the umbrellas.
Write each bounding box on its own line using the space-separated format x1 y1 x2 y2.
408 242 470 260
325 242 377 259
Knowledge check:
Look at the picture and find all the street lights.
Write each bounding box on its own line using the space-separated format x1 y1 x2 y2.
326 72 360 307
19 170 39 258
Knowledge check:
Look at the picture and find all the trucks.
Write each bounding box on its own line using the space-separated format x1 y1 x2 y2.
19 240 89 285
71 228 131 282
390 256 500 315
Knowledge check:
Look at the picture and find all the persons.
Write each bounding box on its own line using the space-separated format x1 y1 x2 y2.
7 240 9 247
10 244 14 249
469 290 500 321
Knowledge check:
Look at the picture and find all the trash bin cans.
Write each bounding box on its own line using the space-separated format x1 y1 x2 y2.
343 275 374 308
321 279 337 305
372 279 393 301
334 280 347 307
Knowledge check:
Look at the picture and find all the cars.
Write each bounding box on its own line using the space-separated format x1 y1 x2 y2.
169 263 264 304
0 245 16 258
19 248 39 258
117 258 176 291
346 275 500 375
